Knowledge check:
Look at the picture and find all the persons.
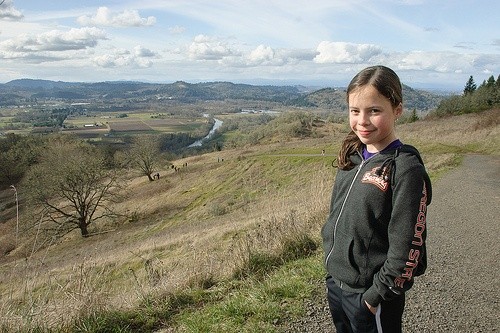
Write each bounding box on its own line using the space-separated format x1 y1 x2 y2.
321 65 432 333
